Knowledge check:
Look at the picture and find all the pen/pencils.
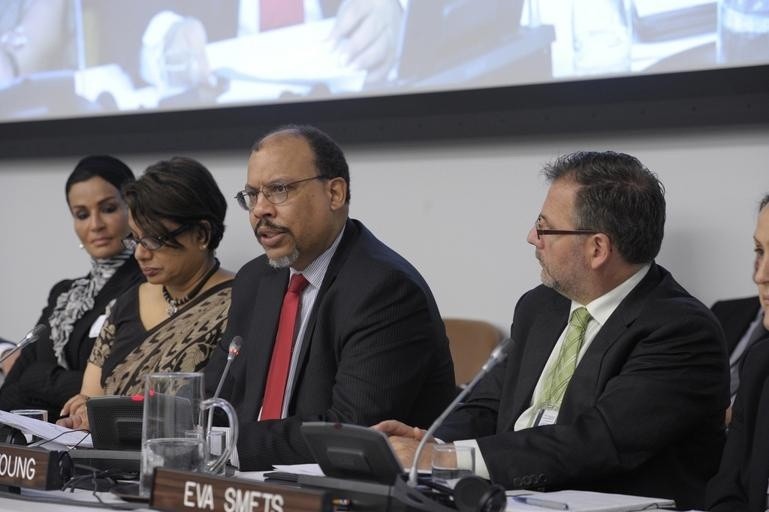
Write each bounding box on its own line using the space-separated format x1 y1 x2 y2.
512 496 567 511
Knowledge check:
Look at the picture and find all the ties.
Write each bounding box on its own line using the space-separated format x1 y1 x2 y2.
259 274 309 420
535 307 593 413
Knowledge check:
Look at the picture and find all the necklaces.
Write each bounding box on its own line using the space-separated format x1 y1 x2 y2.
164 256 221 304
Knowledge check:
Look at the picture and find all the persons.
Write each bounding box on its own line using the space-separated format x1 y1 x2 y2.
369 152 728 490
701 195 769 511
0 2 76 86
58 157 238 436
171 127 455 472
0 157 146 444
81 1 403 90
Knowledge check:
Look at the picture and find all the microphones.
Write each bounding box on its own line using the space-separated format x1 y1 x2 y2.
205 335 244 475
1 324 49 361
407 339 517 489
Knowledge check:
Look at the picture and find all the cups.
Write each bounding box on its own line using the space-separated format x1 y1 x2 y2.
429 444 474 488
138 371 236 498
11 410 47 439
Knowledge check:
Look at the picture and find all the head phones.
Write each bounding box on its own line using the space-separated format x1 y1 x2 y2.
392 474 506 512
28 444 73 482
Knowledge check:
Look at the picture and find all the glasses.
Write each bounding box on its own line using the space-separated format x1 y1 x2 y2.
533 216 612 256
233 173 322 211
119 226 185 252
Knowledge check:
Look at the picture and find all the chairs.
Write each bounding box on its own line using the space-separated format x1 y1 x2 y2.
443 317 502 386
